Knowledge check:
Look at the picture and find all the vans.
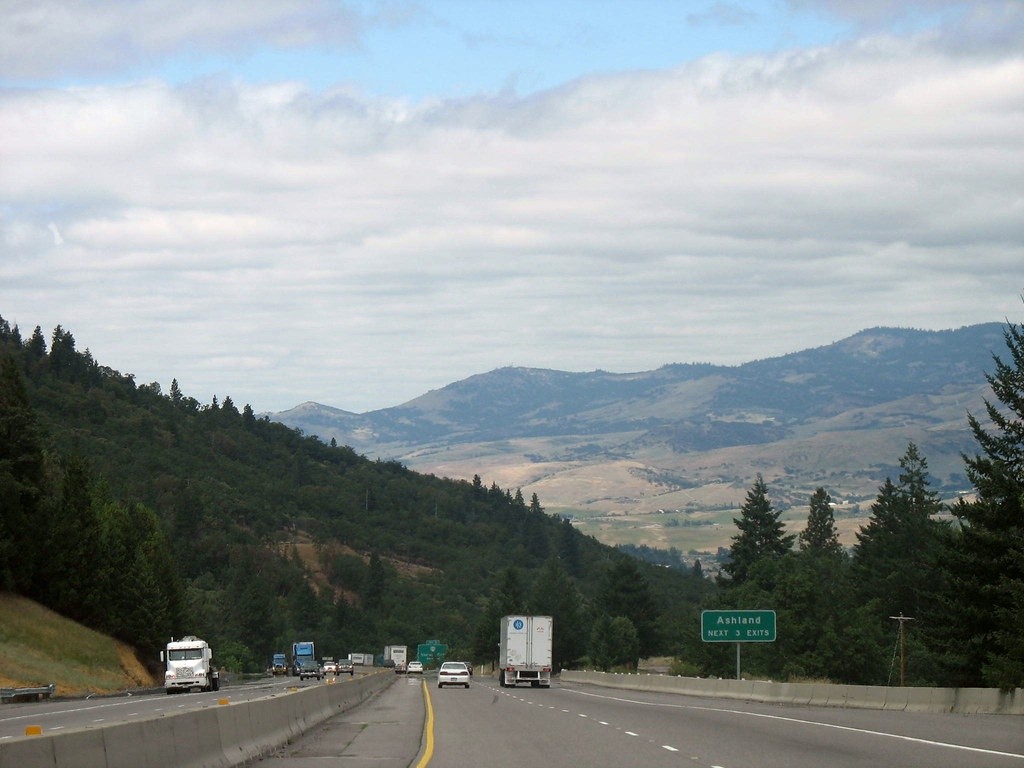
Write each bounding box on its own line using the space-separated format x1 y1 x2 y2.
322 662 337 675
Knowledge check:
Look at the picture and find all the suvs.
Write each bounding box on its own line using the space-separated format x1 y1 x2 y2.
336 658 355 676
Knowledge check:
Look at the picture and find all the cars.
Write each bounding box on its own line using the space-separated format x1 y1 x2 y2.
406 661 424 674
318 665 324 679
298 661 321 680
465 661 473 675
436 661 472 689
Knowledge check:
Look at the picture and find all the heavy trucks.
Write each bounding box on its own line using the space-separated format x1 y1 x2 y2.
160 636 220 695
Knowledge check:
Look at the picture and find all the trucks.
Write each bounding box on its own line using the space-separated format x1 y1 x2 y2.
272 654 289 676
291 642 318 676
384 644 408 675
497 614 552 688
